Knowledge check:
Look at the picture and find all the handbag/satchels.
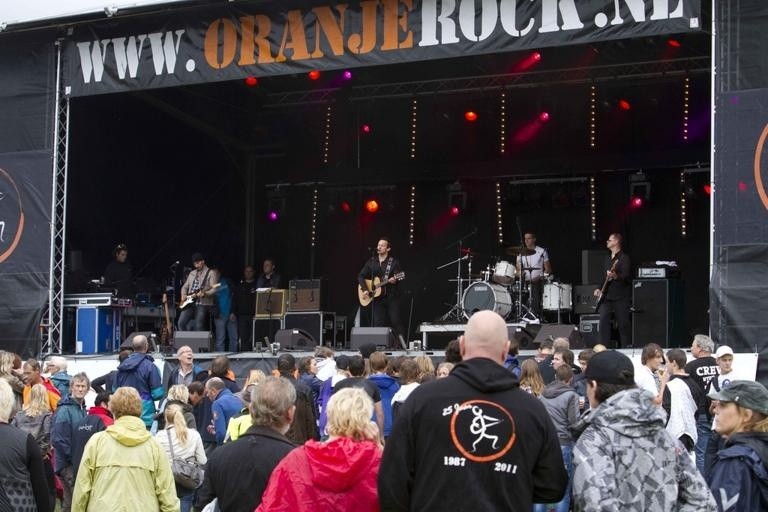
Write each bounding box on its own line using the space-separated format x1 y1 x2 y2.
172 458 204 489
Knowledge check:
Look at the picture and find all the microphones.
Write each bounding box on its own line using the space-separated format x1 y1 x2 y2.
169 260 180 269
538 253 544 264
368 247 378 252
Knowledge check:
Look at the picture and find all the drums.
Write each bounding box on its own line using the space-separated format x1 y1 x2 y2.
543 282 572 310
493 261 516 285
480 262 495 280
462 281 512 321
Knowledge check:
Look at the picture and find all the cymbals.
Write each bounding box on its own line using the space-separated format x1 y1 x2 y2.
506 247 536 256
523 267 540 272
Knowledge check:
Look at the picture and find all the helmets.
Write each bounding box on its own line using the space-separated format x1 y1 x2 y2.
695 334 714 353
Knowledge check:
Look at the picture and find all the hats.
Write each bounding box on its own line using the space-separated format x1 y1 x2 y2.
707 381 768 414
192 253 203 262
716 345 734 358
573 350 633 384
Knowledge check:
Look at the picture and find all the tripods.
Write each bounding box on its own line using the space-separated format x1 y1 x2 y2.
439 261 467 323
519 270 549 323
506 280 540 323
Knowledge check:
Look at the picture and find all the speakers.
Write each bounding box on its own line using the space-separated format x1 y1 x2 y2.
255 288 289 318
581 249 611 286
288 279 321 312
632 278 681 348
532 324 587 349
173 330 212 353
120 331 153 353
349 326 402 350
274 327 317 352
507 326 539 350
573 284 602 315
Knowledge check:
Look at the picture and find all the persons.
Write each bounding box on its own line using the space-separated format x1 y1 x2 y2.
0 232 767 512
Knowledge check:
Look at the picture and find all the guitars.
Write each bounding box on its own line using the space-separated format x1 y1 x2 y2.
595 259 618 311
179 283 221 309
358 272 405 306
162 293 176 347
